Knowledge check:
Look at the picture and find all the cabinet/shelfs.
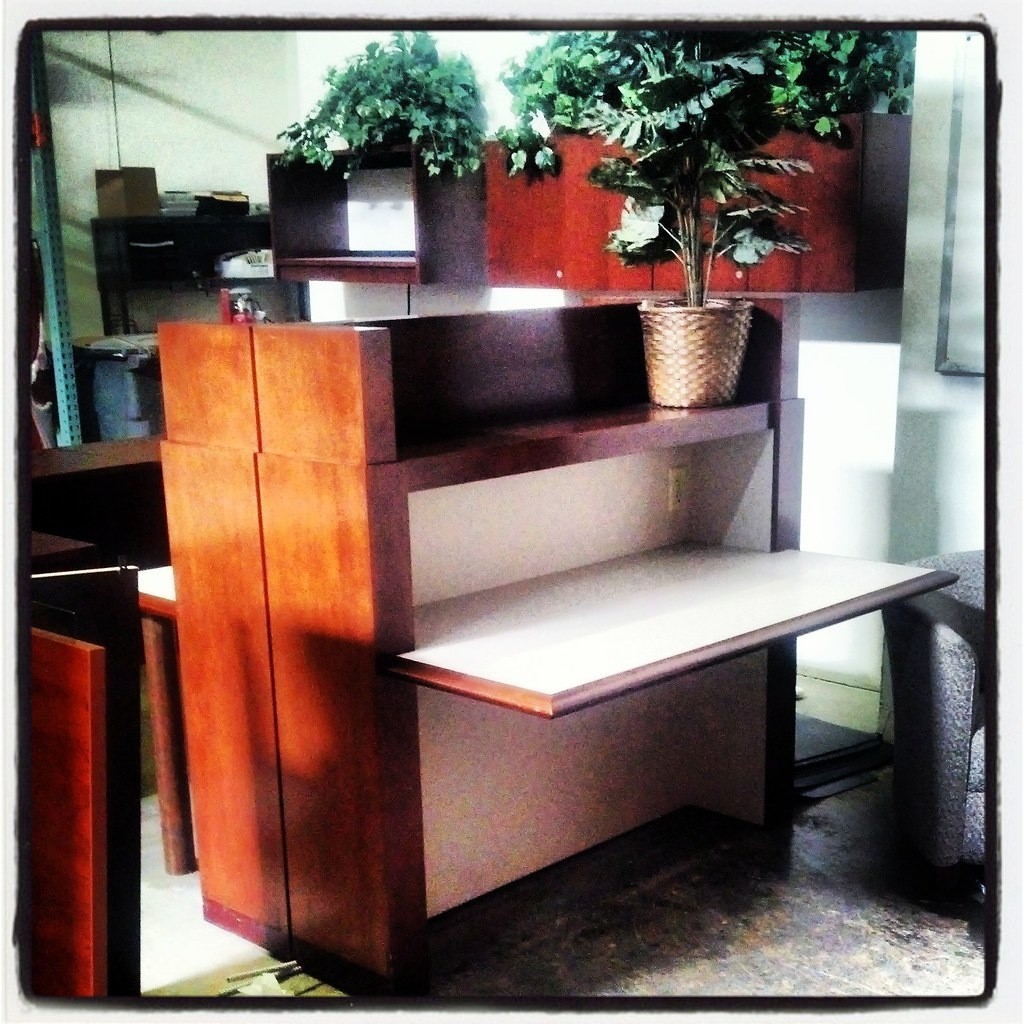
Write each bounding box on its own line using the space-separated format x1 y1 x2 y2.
262 112 911 296
137 297 960 995
31 566 166 995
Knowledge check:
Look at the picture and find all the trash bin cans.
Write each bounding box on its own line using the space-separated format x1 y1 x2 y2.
72 331 162 441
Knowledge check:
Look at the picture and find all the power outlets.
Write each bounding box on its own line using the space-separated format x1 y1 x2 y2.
667 468 688 513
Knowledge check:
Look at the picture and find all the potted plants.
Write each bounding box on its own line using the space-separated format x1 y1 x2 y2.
276 28 916 177
584 42 811 412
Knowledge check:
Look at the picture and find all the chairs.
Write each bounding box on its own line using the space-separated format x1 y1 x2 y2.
882 547 987 894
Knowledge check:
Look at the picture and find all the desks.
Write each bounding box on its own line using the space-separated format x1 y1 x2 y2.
28 420 165 563
89 205 270 333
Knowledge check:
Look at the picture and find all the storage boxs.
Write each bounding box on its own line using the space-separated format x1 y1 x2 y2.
95 166 160 215
